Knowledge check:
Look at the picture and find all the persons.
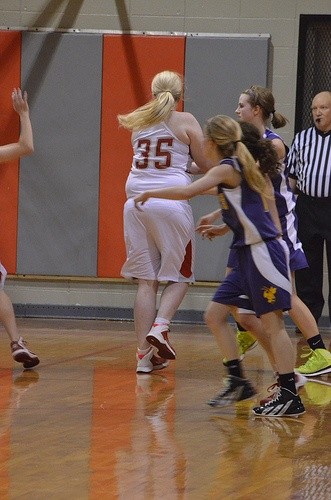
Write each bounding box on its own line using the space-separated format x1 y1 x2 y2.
0 87 40 368
284 91 330 333
116 71 213 374
131 113 307 415
198 86 330 379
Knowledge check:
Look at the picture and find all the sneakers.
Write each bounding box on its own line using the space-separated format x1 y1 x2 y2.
294 344 331 377
11 336 40 369
252 386 306 417
136 346 170 372
260 373 308 406
223 328 258 366
147 322 177 360
206 375 258 409
